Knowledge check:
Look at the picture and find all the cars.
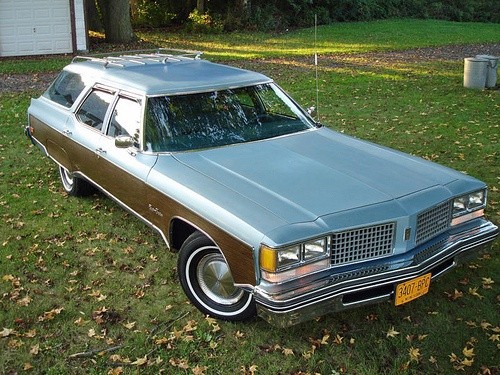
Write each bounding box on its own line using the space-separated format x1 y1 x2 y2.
25 49 500 328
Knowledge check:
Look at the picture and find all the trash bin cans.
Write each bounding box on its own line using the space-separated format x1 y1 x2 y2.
463 54 500 92
